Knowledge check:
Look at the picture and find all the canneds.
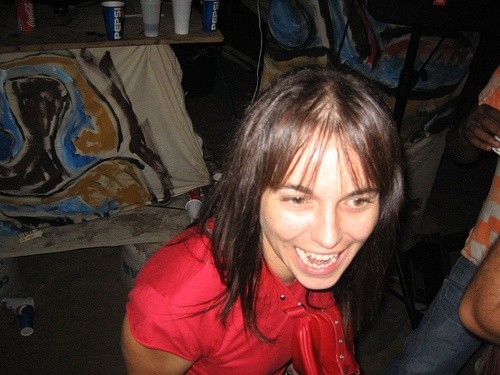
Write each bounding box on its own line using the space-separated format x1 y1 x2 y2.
16 0 35 32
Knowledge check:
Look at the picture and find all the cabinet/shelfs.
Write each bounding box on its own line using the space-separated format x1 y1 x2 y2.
0 0 229 261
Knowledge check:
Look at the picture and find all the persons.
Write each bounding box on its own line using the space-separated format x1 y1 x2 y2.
122 67 406 375
380 66 500 374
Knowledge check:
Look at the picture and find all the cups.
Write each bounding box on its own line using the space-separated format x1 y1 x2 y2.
202 0 220 33
185 199 204 220
171 0 192 35
101 0 125 41
213 172 223 186
140 0 162 38
